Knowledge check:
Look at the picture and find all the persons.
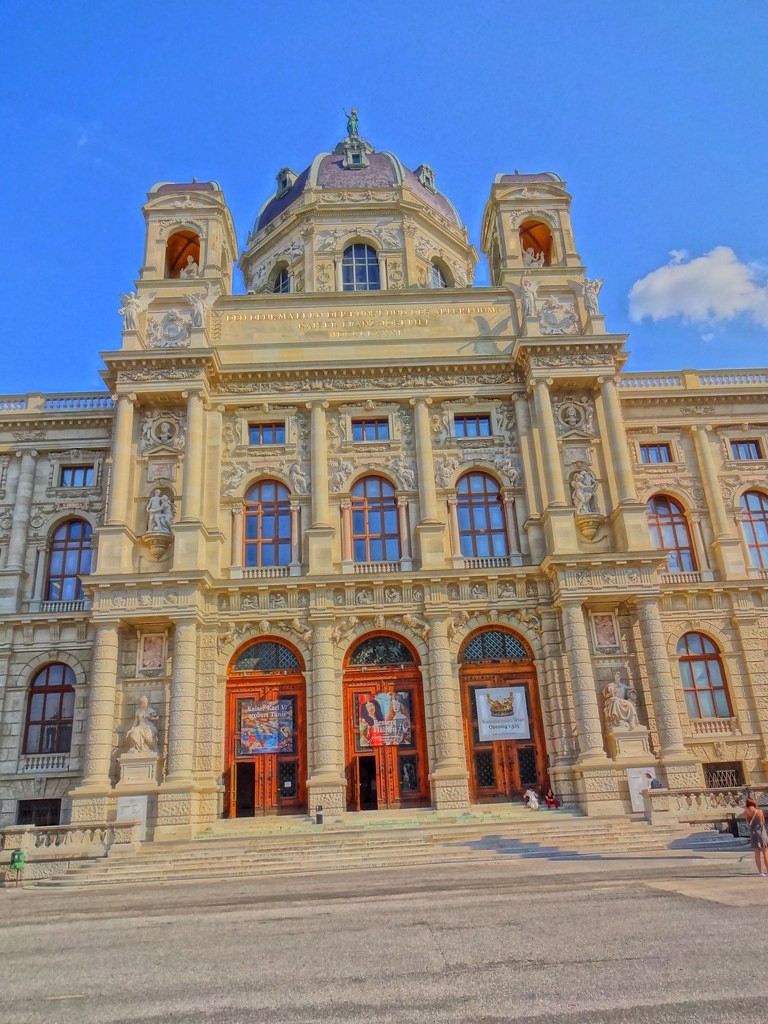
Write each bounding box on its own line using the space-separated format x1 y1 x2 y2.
571 470 598 514
521 238 539 267
180 255 198 279
581 278 599 315
125 292 139 328
140 410 160 440
146 489 172 532
158 423 173 438
218 582 539 610
126 695 160 753
602 671 639 730
744 798 768 877
523 788 540 811
563 407 580 425
190 292 205 326
544 787 559 811
521 272 536 316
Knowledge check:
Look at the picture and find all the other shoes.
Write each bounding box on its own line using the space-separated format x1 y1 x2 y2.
759 873 766 877
